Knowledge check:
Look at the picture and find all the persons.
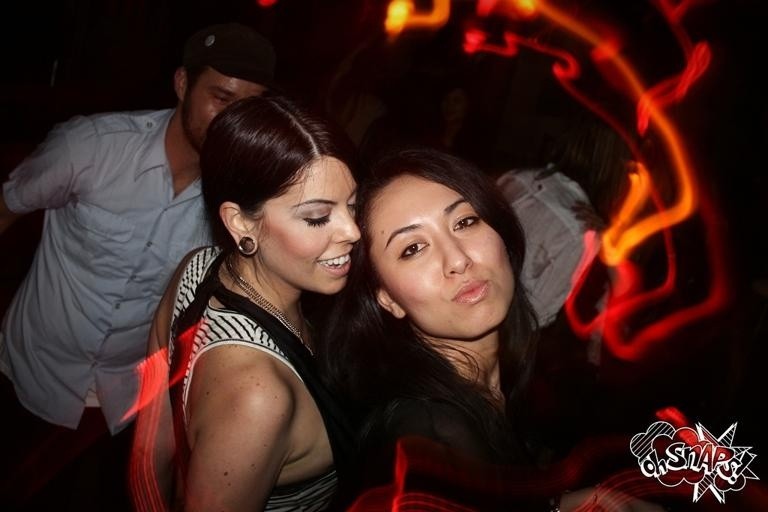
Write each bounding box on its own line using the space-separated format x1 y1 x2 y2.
427 75 476 153
291 148 682 510
468 159 639 493
123 91 365 509
1 21 279 509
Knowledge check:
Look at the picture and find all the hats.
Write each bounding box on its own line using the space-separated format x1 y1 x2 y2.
181 22 282 91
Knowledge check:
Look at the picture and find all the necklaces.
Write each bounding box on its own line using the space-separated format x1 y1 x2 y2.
228 255 316 359
